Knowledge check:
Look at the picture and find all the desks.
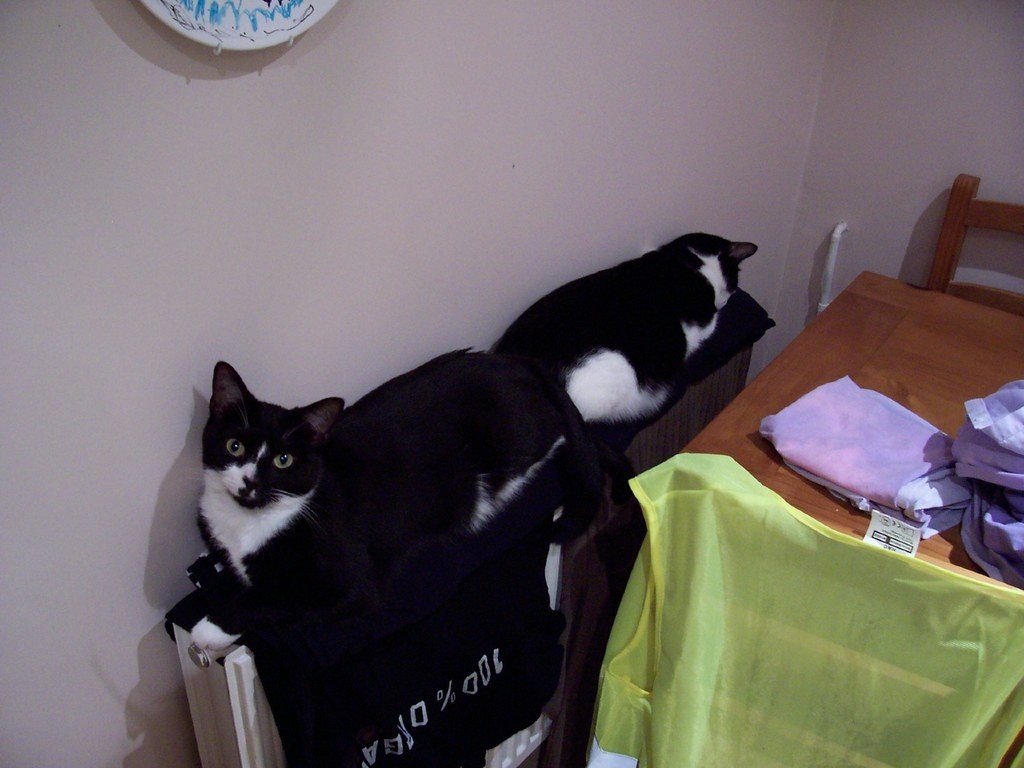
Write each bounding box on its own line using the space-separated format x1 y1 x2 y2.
679 272 1024 595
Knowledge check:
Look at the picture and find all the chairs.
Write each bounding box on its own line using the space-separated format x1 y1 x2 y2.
924 174 1024 317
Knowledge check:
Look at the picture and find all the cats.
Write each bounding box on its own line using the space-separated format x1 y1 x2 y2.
186 360 565 651
495 230 759 426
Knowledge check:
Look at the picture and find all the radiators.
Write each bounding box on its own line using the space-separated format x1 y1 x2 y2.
173 342 752 768
